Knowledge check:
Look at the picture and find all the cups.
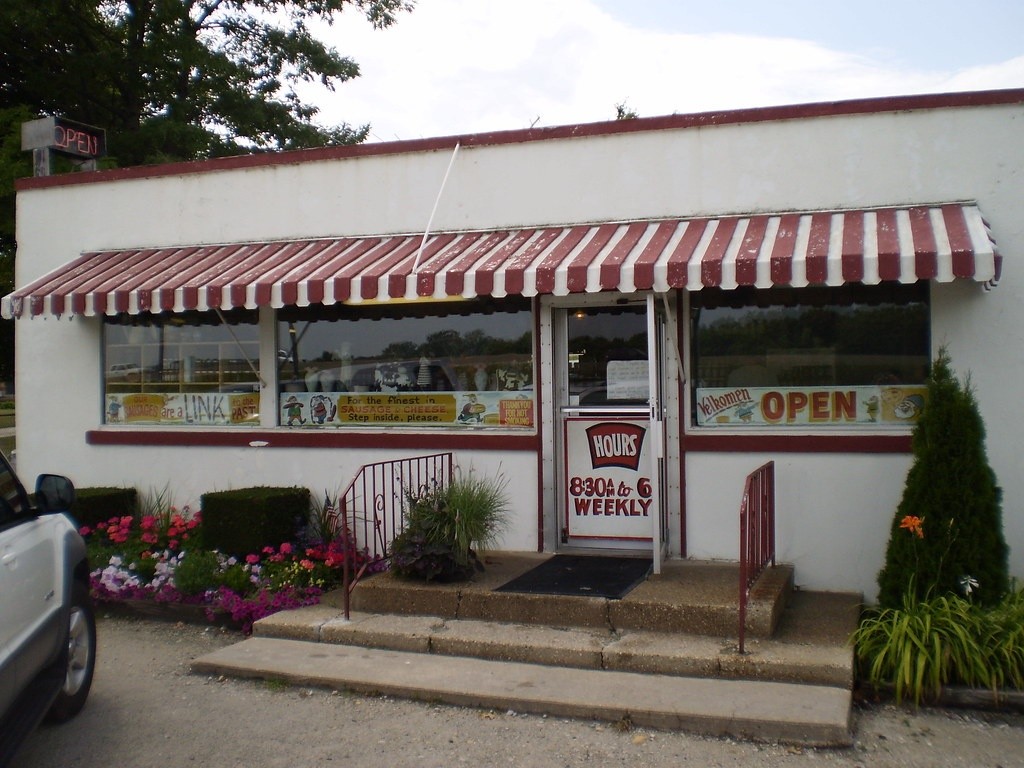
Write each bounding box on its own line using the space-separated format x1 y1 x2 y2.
354 386 369 392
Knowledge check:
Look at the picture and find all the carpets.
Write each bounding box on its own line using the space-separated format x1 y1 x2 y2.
491 553 653 600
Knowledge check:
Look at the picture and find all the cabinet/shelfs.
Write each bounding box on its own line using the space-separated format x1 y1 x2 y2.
105 338 259 393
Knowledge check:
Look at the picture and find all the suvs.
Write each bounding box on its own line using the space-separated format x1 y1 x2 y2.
0 444 98 768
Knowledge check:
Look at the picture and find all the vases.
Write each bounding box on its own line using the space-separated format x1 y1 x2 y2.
284 366 488 395
433 550 478 584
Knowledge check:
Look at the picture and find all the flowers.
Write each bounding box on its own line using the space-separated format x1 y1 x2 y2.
383 455 512 584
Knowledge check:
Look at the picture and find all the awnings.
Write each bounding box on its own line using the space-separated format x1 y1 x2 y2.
1 199 1004 320
